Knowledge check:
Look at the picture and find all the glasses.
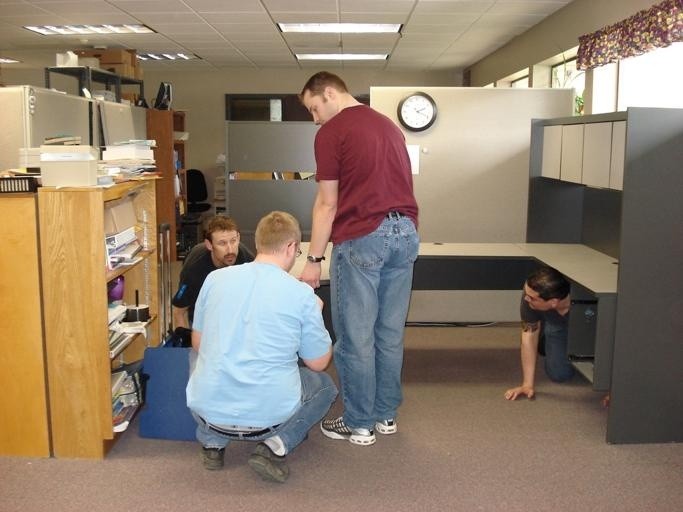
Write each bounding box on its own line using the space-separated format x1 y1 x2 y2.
288 242 303 257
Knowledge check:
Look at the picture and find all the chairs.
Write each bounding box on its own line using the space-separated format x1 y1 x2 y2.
183 170 210 241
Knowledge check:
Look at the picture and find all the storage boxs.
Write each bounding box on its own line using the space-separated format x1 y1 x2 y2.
75 46 144 80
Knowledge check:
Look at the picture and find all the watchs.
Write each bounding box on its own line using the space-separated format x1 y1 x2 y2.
305 255 326 262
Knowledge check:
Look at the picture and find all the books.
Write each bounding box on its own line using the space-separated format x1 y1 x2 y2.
105 226 142 352
19 133 164 187
111 360 144 432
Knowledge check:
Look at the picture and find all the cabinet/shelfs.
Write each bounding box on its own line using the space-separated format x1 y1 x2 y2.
46 66 143 119
522 107 683 442
147 108 187 262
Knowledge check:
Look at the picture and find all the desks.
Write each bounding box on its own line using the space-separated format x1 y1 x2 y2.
291 241 618 395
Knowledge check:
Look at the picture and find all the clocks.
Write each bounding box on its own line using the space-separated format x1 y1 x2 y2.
398 93 438 131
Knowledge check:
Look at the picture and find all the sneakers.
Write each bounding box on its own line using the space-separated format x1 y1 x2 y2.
248 443 290 483
374 417 399 435
321 417 377 446
202 447 225 470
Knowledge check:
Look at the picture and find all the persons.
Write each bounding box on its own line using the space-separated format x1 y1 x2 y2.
173 213 254 332
503 267 609 407
185 211 339 484
299 70 419 445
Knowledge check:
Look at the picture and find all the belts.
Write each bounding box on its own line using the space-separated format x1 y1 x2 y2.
386 211 406 219
198 417 282 437
